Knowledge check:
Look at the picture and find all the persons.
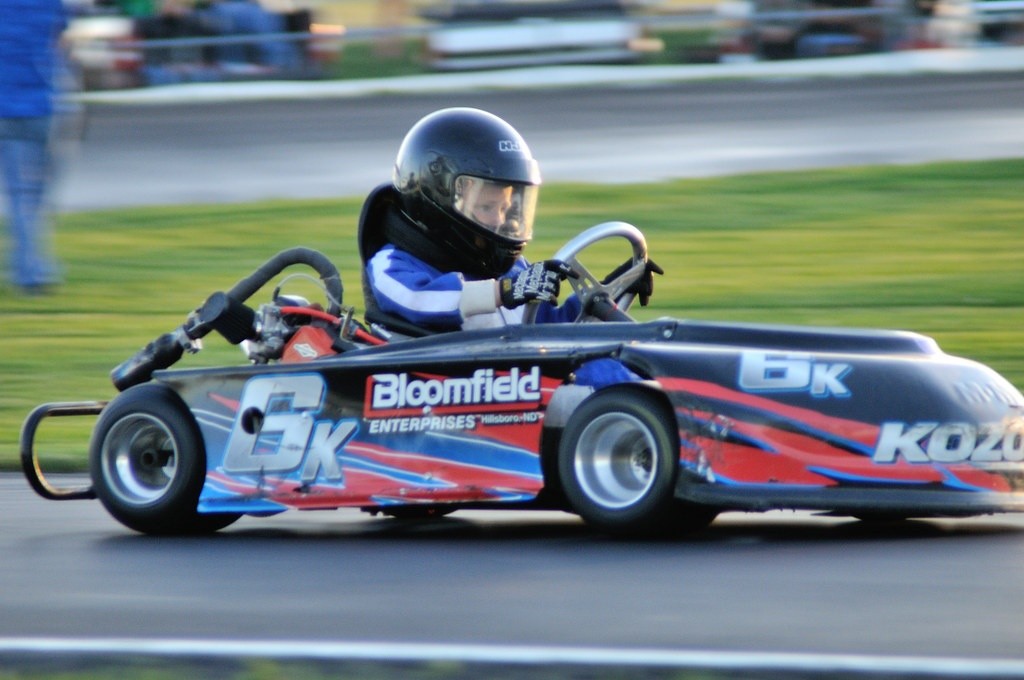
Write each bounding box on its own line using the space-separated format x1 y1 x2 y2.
0 1 71 293
361 107 663 340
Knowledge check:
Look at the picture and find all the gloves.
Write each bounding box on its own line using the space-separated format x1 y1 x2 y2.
599 255 664 306
499 260 579 309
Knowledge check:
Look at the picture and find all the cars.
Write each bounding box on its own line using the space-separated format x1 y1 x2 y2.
419 2 649 74
713 1 934 63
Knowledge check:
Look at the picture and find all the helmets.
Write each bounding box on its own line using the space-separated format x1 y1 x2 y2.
394 106 543 276
